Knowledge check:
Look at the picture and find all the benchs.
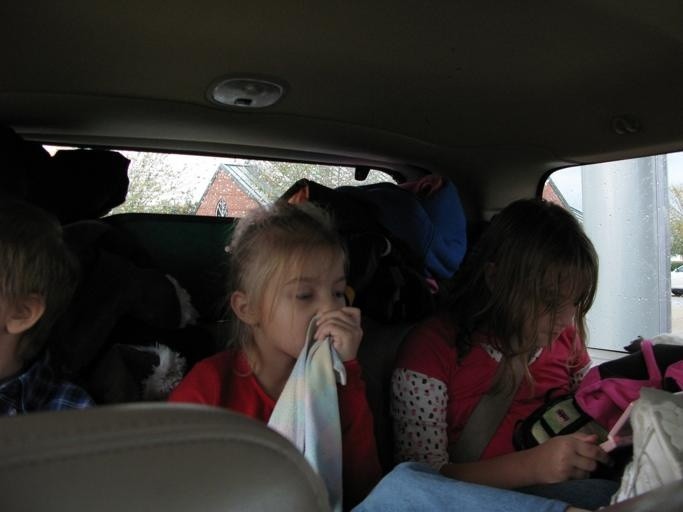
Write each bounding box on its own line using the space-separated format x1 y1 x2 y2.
83 213 252 395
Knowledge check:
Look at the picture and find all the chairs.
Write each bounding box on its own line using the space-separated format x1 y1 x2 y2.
0 398 331 511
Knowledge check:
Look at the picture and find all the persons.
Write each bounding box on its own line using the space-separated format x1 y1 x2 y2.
0 202 99 418
387 199 631 508
169 201 596 512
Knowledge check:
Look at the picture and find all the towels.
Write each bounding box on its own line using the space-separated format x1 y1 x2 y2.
269 316 349 511
